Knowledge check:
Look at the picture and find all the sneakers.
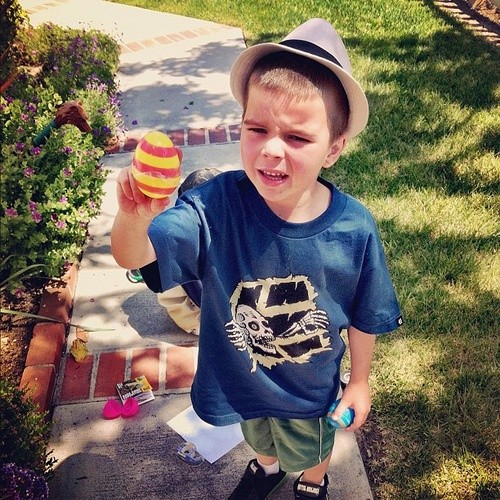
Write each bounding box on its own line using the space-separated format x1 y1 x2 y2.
292 471 331 500
227 458 288 500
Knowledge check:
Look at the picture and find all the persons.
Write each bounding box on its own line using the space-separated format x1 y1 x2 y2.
110 19 405 500
117 379 143 397
157 166 228 335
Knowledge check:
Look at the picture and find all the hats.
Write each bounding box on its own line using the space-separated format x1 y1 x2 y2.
229 18 369 140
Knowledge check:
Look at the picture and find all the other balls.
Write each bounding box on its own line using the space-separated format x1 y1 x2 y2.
327 398 354 429
131 131 181 199
